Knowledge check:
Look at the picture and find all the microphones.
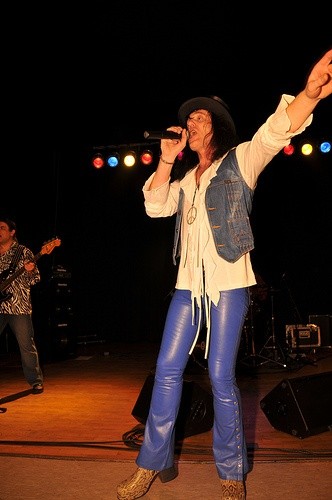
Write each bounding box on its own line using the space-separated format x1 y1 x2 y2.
143 131 190 140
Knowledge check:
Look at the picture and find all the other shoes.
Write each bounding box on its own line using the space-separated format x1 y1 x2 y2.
32 384 44 394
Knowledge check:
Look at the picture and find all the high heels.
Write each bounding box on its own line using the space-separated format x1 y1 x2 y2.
217 478 247 500
115 462 178 500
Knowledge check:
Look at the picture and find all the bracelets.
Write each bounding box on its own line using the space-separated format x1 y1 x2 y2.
160 155 176 165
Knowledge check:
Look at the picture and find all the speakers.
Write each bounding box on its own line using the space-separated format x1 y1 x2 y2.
131 375 214 439
309 315 332 349
259 371 332 439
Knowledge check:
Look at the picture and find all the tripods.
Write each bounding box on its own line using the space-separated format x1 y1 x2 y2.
239 287 288 368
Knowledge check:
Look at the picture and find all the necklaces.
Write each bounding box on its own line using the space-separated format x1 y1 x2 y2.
185 162 213 225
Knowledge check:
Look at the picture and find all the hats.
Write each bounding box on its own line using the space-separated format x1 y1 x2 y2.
177 94 237 143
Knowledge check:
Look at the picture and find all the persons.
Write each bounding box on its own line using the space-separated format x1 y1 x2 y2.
115 48 332 500
0 218 45 394
